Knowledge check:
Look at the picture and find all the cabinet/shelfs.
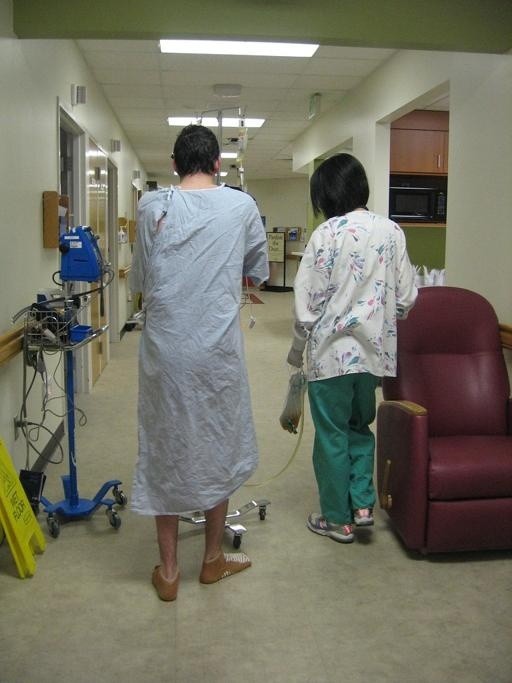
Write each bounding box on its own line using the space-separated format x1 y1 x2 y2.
390 128 444 173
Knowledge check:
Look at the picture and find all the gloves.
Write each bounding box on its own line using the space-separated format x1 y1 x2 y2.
287 321 309 368
280 373 307 434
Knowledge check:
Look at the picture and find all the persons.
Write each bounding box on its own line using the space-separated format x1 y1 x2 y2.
286 154 418 542
125 122 270 602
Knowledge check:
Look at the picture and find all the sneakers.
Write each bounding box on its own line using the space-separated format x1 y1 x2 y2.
306 512 354 544
351 506 375 526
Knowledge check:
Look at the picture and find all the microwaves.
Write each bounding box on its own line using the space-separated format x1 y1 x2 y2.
389 183 447 222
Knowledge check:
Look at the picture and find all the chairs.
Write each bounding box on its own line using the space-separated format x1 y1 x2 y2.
372 282 512 560
